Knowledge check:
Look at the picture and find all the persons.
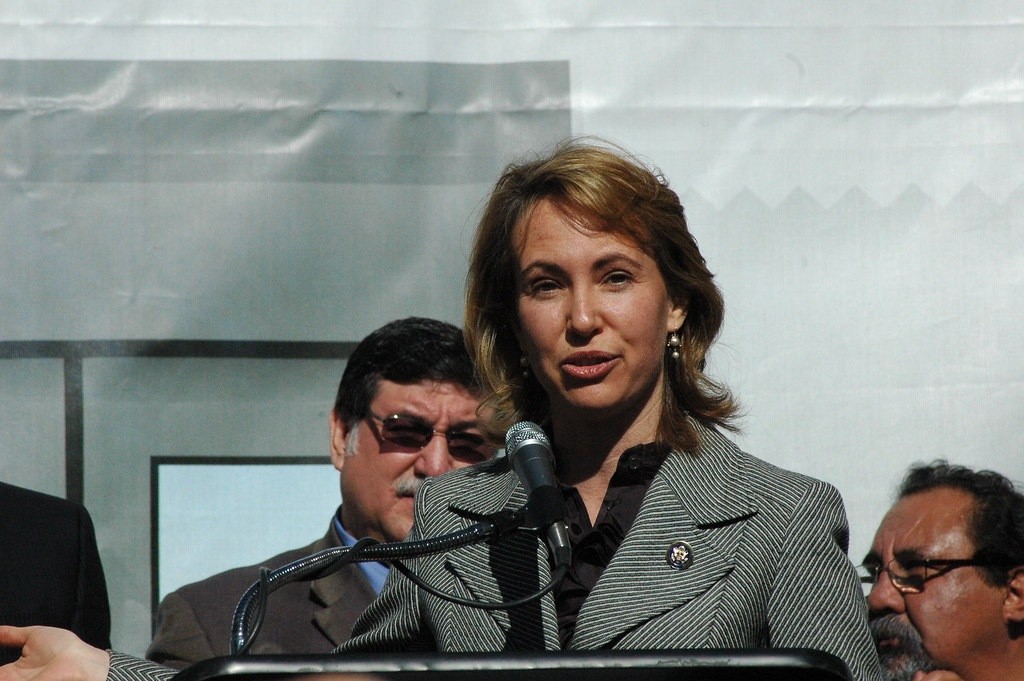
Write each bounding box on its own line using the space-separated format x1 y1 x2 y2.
862 457 1024 681
145 315 505 672
0 133 884 681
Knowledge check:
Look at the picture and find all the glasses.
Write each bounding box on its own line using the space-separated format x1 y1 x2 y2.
353 408 499 463
853 554 991 599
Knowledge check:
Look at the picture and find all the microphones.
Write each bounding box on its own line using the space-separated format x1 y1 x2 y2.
505 422 572 569
